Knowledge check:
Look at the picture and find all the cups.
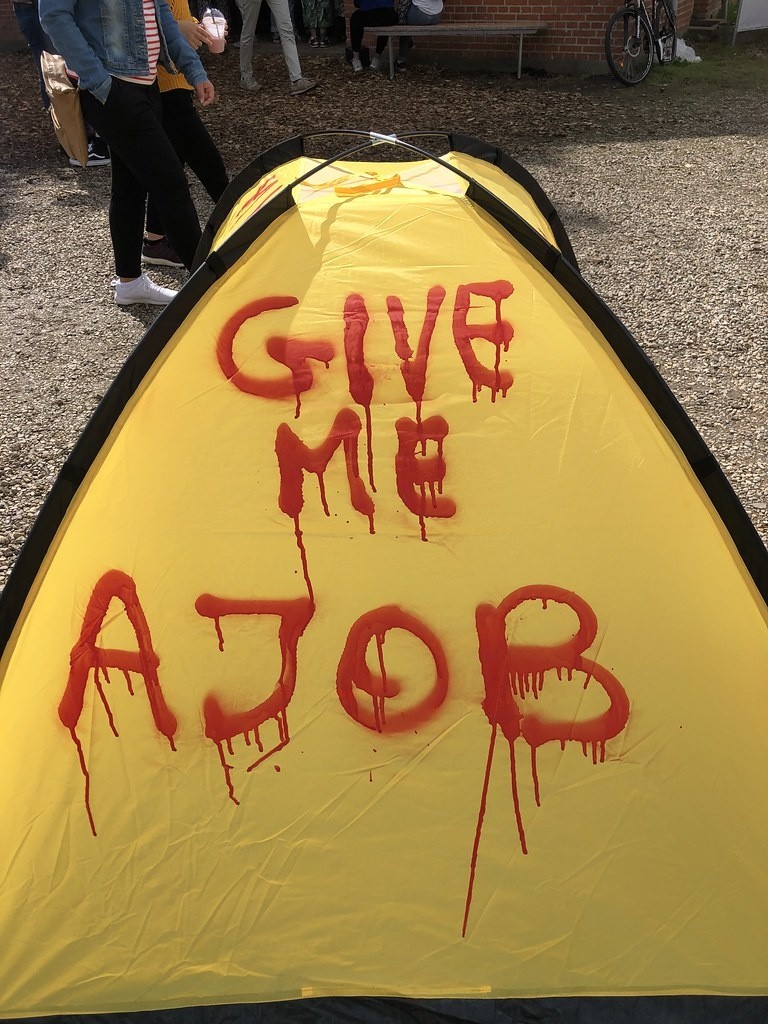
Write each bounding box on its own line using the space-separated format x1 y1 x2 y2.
201 8 226 52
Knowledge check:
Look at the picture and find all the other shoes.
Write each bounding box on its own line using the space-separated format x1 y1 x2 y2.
351 58 363 71
272 34 281 44
369 56 380 71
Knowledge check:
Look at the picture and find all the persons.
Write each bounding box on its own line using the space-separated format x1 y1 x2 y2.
36 0 215 303
140 0 230 266
351 0 395 72
397 0 444 72
300 1 331 48
271 0 301 43
12 1 110 165
235 1 319 96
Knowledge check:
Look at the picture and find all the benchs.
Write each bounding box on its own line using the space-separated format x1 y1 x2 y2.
364 20 550 82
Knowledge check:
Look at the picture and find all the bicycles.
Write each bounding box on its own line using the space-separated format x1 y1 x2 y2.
605 0 678 85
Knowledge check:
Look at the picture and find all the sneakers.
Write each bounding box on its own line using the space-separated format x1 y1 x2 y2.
394 59 407 72
408 36 416 50
320 36 331 48
69 136 111 167
141 236 185 268
240 74 264 91
114 273 179 305
307 35 319 48
289 77 319 96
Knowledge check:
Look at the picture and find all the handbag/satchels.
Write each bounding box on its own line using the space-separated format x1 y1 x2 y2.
40 50 89 169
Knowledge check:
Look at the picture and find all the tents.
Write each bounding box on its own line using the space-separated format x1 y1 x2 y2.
3 134 767 1022
